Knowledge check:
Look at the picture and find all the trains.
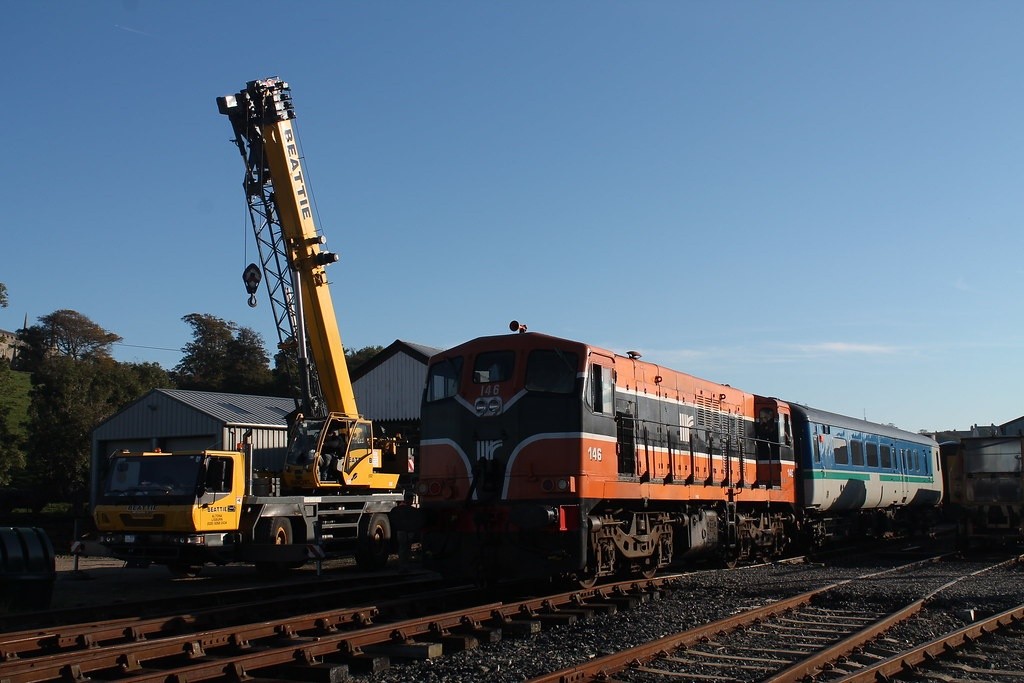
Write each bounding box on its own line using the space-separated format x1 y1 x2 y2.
959 423 1024 553
418 316 965 592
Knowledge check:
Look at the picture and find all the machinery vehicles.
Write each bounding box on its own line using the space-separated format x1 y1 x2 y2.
72 74 421 574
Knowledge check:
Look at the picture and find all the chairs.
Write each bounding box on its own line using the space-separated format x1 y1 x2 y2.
145 468 184 493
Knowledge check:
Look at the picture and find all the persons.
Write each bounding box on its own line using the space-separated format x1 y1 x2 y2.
314 429 344 481
